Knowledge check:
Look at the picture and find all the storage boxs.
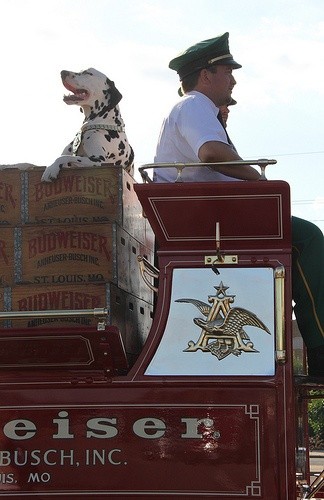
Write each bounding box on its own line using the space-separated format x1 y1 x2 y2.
2 166 158 357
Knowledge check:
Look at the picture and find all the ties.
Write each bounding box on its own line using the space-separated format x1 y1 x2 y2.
218 111 239 155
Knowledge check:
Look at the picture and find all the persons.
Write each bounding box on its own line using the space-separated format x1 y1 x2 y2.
177 84 237 126
150 30 323 378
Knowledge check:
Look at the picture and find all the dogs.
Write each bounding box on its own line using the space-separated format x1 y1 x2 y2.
0 68 134 183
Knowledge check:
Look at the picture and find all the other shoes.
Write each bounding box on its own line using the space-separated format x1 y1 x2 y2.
306 344 324 384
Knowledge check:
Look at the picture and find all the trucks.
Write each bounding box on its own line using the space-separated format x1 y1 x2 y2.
0 159 323 498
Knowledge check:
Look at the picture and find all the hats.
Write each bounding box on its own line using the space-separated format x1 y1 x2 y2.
168 32 243 79
178 87 238 106
